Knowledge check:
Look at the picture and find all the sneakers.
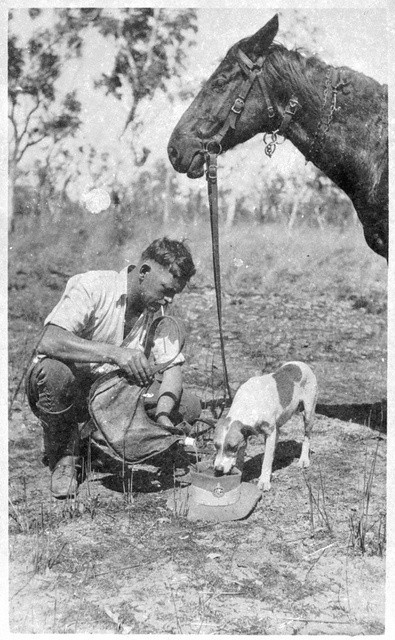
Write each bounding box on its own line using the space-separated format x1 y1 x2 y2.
49 455 87 498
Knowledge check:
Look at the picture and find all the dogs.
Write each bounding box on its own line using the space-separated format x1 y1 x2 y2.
193 360 319 493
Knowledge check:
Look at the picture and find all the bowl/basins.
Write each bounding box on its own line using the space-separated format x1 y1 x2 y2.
190 466 242 507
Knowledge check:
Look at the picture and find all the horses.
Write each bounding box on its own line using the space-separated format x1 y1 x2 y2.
166 11 389 260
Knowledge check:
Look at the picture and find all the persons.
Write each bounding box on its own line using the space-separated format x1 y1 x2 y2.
24 235 202 500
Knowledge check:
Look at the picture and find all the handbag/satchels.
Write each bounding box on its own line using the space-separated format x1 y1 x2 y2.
85 314 194 466
